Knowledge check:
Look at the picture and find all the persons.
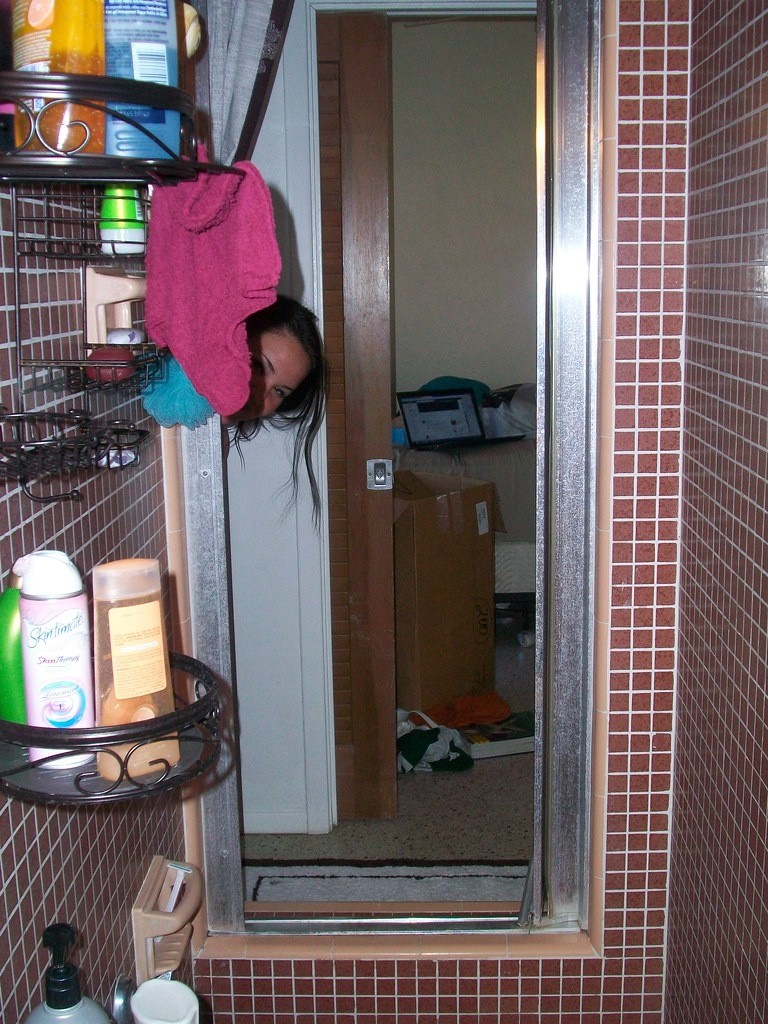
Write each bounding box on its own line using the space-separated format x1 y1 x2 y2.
219 292 330 539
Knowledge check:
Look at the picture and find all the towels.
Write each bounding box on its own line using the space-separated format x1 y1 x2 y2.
144 139 281 418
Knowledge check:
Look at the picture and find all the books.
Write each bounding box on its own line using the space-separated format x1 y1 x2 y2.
453 708 535 760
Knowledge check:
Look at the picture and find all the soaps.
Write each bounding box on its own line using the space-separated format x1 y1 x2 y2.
83 346 136 383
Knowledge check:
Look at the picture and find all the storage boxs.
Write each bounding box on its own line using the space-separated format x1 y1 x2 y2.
393 470 508 712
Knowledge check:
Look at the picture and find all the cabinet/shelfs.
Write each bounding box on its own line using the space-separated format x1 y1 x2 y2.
0 68 250 503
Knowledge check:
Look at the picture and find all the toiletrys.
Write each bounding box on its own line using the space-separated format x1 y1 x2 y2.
26 924 111 1024
102 0 178 157
0 563 31 724
12 549 98 771
91 556 182 782
11 0 105 154
99 184 148 255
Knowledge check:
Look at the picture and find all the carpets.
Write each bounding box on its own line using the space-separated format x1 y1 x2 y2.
241 858 531 901
